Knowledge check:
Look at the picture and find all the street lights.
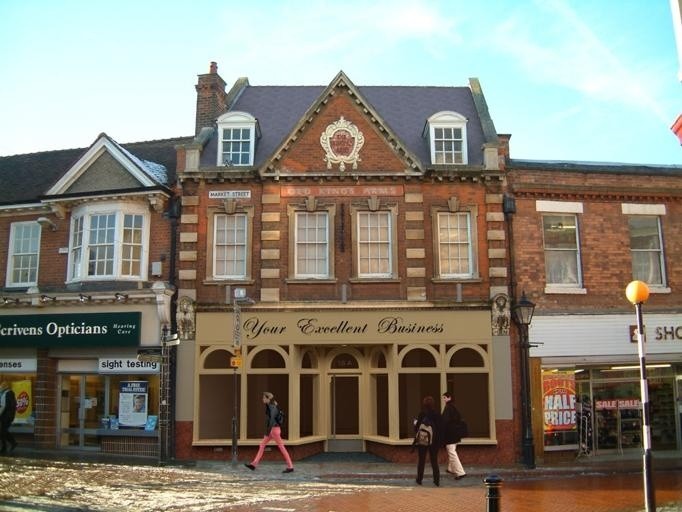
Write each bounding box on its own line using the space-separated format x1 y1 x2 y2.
624 280 658 512
509 290 544 469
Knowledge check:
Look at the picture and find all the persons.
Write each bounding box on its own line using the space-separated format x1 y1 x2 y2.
411 391 467 486
0 380 18 453
244 390 295 474
133 394 145 413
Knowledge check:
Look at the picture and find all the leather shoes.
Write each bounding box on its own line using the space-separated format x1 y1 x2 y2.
446 469 454 474
455 474 467 480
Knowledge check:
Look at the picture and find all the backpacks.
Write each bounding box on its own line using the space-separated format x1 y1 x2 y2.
415 416 433 445
277 410 285 424
447 403 467 438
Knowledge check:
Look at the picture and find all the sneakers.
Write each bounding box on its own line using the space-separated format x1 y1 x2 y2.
433 480 440 487
416 479 422 484
245 464 255 470
283 468 293 473
